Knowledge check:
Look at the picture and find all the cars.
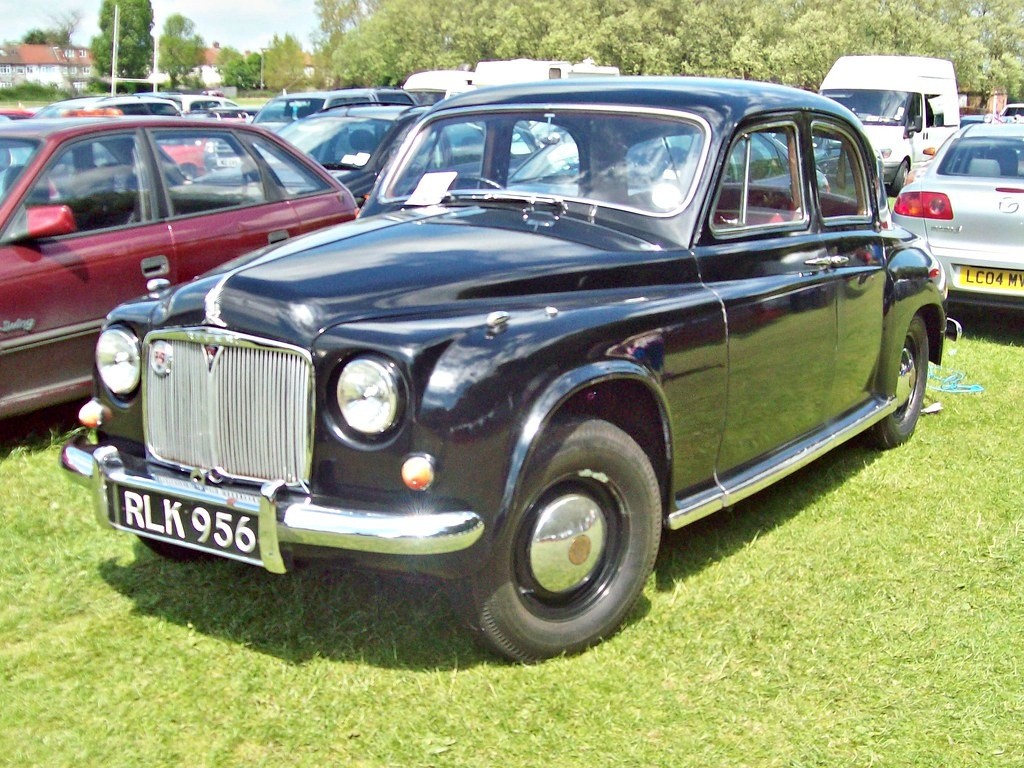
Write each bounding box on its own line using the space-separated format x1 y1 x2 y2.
0 115 363 421
997 103 1024 124
54 75 961 665
959 107 991 129
0 88 831 218
888 122 1024 321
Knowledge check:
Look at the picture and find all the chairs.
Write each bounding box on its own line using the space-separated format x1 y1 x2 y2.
348 129 379 158
968 156 1001 179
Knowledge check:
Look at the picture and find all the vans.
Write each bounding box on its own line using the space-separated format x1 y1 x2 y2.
808 56 961 200
404 58 621 115
244 85 419 157
